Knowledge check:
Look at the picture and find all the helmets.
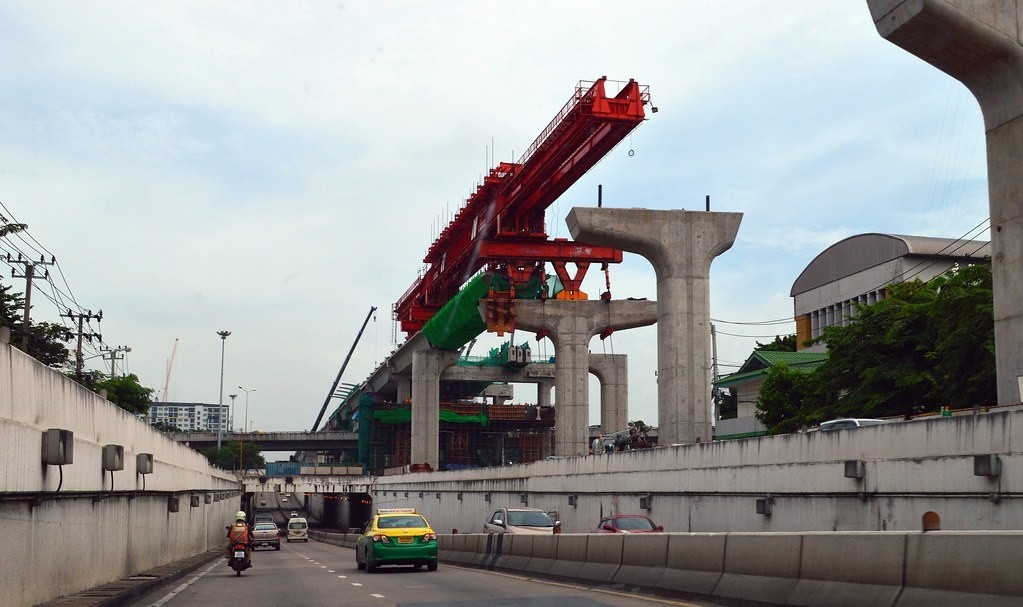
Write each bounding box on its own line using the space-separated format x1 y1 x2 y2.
236 511 246 523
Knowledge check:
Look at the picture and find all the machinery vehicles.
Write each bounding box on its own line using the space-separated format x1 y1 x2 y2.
296 305 378 461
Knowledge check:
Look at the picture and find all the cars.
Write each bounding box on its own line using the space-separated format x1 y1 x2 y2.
354 508 439 573
291 511 298 517
482 507 556 536
283 497 288 502
261 501 266 506
591 514 664 533
286 493 291 497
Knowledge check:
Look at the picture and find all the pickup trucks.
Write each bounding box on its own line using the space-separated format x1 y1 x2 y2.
250 523 282 550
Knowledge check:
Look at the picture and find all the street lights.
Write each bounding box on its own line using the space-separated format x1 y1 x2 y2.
229 394 237 433
238 386 256 436
215 330 232 451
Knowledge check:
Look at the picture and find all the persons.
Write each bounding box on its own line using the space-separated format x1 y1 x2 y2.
226 510 255 567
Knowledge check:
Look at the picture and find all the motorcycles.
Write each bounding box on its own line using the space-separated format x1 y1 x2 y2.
225 525 252 577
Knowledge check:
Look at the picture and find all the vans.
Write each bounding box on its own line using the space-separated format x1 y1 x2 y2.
285 518 309 542
254 513 274 528
